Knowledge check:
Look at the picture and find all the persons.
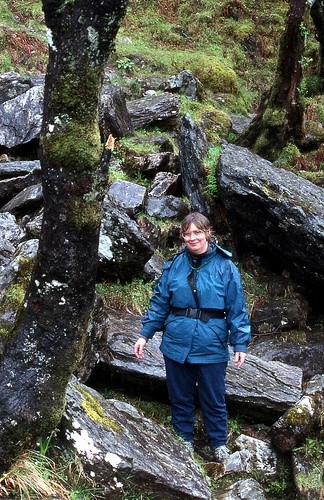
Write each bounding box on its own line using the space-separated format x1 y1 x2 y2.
134 212 252 462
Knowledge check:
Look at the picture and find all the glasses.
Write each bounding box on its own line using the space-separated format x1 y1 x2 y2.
182 230 209 239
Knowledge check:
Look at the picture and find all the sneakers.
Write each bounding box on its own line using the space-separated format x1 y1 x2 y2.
212 444 230 464
181 442 194 460
